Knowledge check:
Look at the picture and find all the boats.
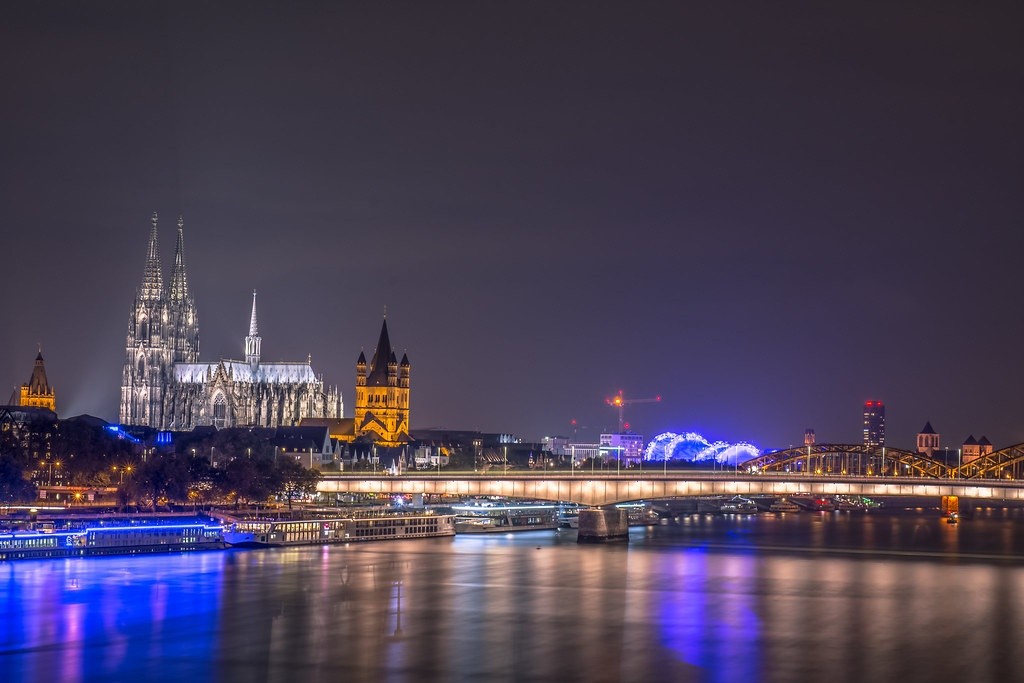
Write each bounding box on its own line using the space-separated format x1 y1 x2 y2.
558 503 661 528
224 515 456 546
0 523 235 560
453 501 560 533
770 498 801 512
720 499 758 514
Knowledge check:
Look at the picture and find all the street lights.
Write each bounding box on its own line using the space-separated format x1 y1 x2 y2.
789 444 792 471
374 448 376 475
572 446 574 476
505 447 506 475
882 447 885 477
618 446 620 475
311 448 313 468
958 449 961 478
735 446 737 477
945 446 949 480
664 446 667 476
438 447 440 477
